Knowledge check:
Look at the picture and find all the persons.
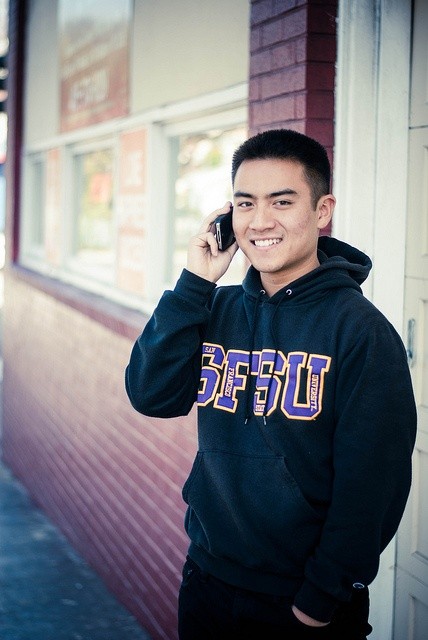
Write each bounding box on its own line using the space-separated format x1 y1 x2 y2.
121 127 419 636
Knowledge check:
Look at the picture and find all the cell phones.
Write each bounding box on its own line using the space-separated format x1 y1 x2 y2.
214 206 237 251
